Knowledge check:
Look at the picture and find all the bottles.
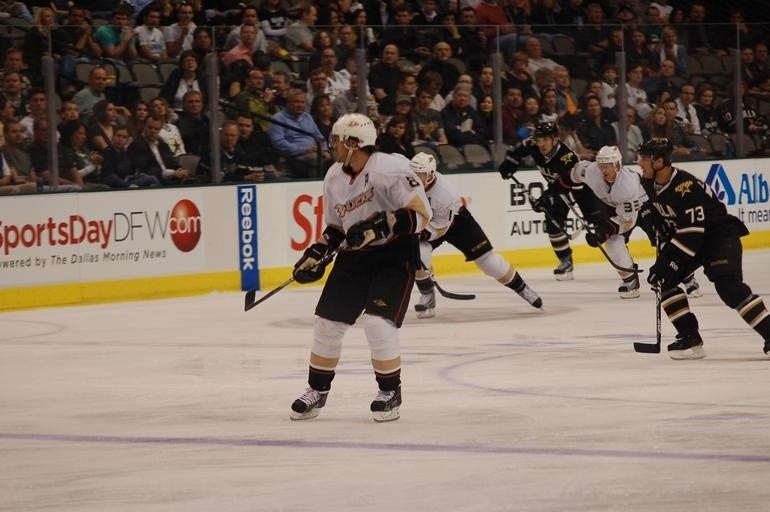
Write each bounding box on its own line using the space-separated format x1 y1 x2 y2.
725 137 732 158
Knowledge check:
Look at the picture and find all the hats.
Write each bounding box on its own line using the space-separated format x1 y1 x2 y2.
394 94 414 104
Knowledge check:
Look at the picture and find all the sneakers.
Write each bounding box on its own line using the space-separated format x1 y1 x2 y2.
668 313 703 351
415 292 436 311
619 264 639 291
554 257 573 274
370 382 402 412
519 285 541 308
685 278 699 294
291 387 327 413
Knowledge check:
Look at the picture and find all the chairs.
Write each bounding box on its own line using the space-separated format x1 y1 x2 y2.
408 142 519 176
67 56 185 110
680 54 769 158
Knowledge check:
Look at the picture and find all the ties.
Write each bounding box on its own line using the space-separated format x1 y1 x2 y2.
685 106 691 123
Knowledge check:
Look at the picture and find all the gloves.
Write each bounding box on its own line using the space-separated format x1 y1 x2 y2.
639 205 670 235
293 244 331 283
499 149 521 180
532 192 554 212
548 175 571 194
346 210 391 251
647 256 679 292
586 219 619 247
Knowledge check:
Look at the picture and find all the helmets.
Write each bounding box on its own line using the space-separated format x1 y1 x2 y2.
534 121 560 145
633 136 672 165
329 113 377 148
595 145 622 176
410 151 437 185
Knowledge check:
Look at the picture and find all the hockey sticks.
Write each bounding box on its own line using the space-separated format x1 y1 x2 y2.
421 260 475 301
244 219 386 312
634 230 663 353
511 175 586 241
561 194 643 274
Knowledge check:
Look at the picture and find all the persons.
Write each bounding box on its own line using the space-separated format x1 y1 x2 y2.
288 112 435 416
498 122 583 276
406 151 542 312
548 144 700 295
1 0 769 197
637 135 770 354
